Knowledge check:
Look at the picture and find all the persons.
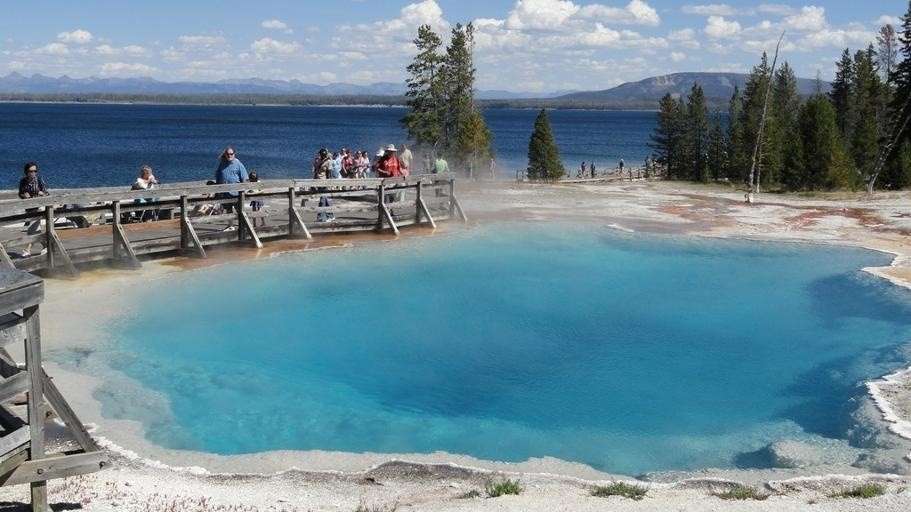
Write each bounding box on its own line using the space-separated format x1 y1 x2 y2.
313 143 413 222
431 151 450 196
619 159 625 174
18 162 50 257
133 165 157 204
198 148 266 232
581 161 586 178
488 158 496 179
645 156 657 167
591 160 595 177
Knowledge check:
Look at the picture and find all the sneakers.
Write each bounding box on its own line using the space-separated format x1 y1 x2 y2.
21 249 31 257
40 247 48 255
223 226 236 232
325 215 335 222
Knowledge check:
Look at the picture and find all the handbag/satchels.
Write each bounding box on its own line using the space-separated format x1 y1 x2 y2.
339 169 348 177
347 169 355 175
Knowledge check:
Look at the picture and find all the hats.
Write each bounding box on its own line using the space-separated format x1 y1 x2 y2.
385 144 398 152
